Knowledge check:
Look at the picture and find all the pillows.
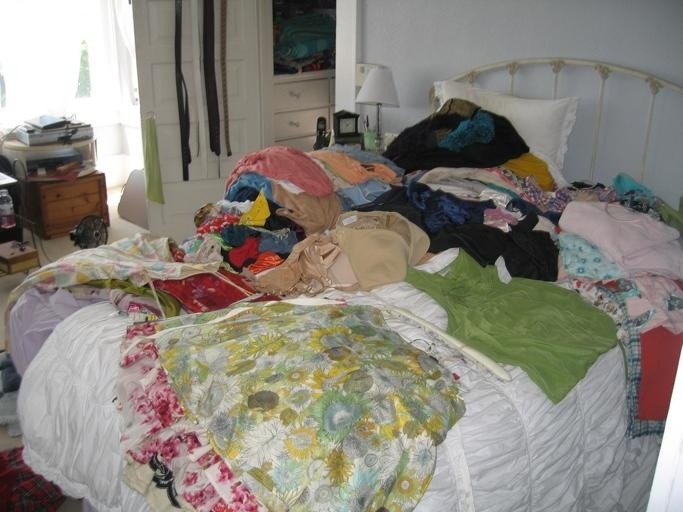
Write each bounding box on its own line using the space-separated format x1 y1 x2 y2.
433 80 580 172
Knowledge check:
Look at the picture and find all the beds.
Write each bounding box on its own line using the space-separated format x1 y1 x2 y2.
7 55 683 511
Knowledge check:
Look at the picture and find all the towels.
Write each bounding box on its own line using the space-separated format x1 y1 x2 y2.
142 118 165 204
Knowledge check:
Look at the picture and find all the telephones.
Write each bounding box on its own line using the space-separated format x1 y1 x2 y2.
316 117 331 147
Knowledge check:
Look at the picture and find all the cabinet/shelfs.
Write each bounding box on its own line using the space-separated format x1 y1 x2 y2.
257 1 337 148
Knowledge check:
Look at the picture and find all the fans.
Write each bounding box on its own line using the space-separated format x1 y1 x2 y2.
69 214 108 251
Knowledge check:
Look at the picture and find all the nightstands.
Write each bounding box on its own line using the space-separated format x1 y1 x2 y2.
312 132 400 153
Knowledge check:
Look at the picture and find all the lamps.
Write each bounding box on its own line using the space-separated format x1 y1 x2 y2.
355 67 400 145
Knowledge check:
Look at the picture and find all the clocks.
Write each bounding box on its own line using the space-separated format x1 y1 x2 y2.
332 109 359 138
